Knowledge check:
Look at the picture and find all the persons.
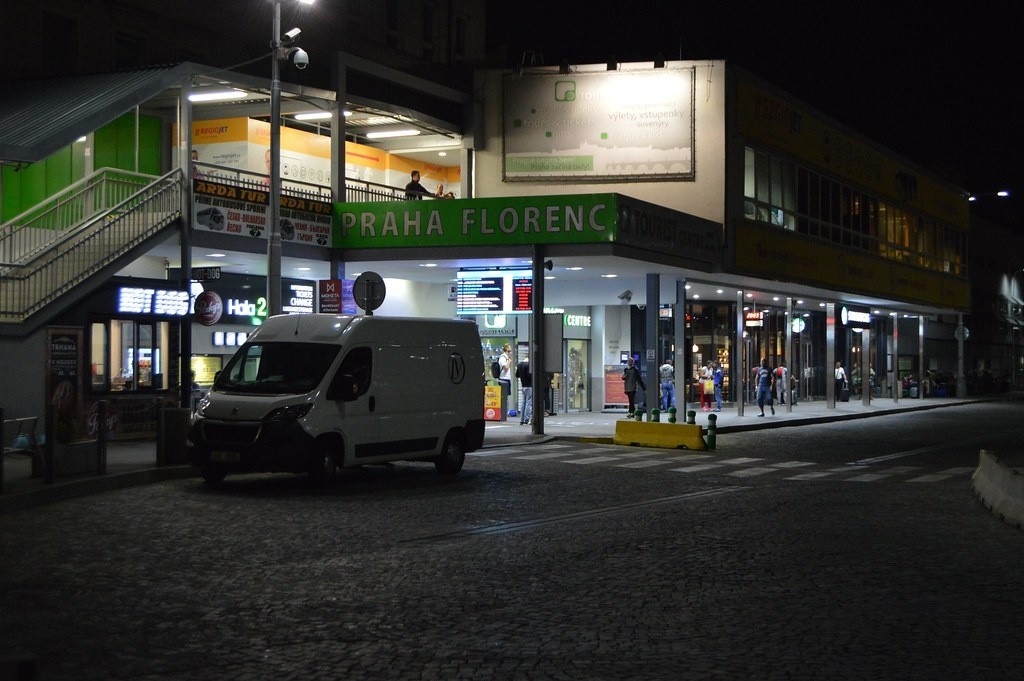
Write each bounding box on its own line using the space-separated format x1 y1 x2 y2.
405 170 439 201
515 353 532 425
660 360 675 413
699 360 724 412
870 363 875 400
773 360 799 405
622 357 645 418
755 358 775 417
543 373 554 417
498 343 512 395
434 184 453 198
190 371 199 389
191 150 203 180
52 368 74 415
935 372 955 384
835 362 848 402
851 363 861 400
902 374 918 390
801 363 814 379
259 150 271 191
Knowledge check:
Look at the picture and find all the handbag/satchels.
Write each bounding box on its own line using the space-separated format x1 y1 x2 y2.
704 378 714 394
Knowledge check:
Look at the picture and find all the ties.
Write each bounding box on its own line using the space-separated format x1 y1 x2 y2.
835 368 839 378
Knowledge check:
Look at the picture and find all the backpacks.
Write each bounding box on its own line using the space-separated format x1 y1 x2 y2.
491 355 507 378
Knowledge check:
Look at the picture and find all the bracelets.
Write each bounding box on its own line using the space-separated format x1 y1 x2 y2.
844 379 847 380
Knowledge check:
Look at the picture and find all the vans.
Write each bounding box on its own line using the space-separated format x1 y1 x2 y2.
188 311 488 489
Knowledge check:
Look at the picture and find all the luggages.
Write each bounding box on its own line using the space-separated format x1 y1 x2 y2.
909 382 918 398
792 380 798 404
840 382 849 402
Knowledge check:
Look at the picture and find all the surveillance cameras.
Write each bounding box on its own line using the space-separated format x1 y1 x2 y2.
284 27 301 40
294 49 309 68
617 289 632 299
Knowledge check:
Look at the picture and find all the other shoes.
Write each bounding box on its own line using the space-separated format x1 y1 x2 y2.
708 408 714 411
772 410 775 415
702 406 708 412
758 413 764 416
627 413 634 418
520 422 528 425
716 408 721 412
